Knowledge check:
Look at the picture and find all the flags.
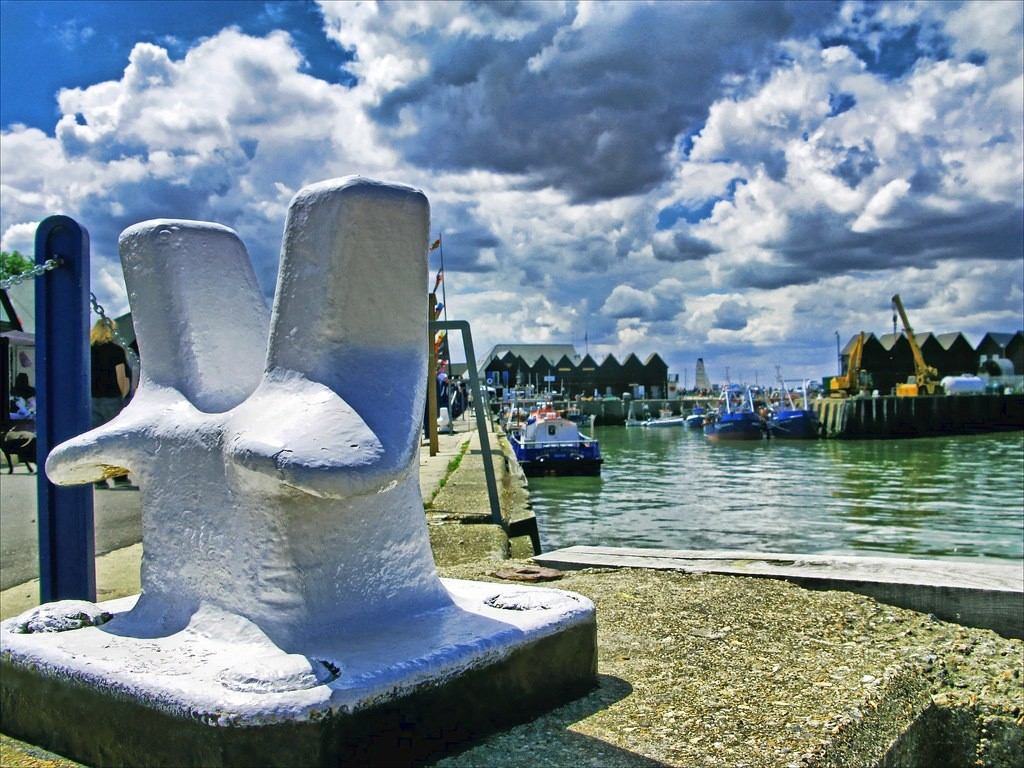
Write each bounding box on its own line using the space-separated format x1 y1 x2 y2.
427 238 448 371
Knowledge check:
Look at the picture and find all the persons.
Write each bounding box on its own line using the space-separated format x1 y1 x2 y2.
87 318 132 489
10 374 36 412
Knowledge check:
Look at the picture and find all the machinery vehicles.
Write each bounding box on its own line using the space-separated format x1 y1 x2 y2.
888 293 947 396
827 330 877 399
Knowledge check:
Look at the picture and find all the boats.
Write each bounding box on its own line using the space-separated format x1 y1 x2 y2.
483 359 826 475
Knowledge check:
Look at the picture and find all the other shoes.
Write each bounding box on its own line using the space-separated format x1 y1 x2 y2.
114 480 131 486
95 483 109 488
